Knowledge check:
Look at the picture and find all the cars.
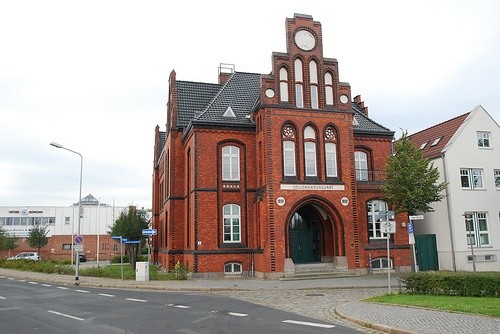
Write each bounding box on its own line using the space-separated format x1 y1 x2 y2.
75 254 87 262
7 252 41 263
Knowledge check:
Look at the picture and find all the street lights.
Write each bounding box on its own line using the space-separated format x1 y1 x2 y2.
50 141 83 280
